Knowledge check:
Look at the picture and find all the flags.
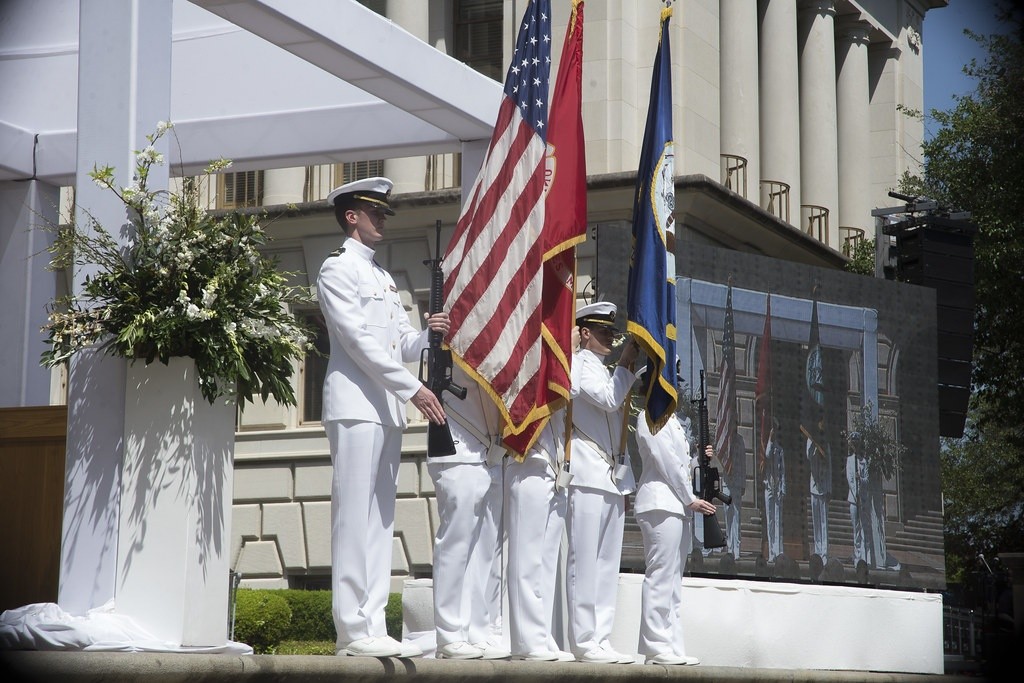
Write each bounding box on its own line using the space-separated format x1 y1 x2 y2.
712 278 830 484
439 0 586 461
627 7 679 433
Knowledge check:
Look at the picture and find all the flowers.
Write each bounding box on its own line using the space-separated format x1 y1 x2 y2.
843 403 904 479
14 120 330 414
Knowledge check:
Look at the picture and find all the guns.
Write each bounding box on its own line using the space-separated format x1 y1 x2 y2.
691 369 732 548
855 450 861 519
418 219 467 458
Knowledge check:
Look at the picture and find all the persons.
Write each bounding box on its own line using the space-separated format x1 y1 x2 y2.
633 354 717 666
428 301 640 663
315 175 453 658
688 549 868 584
719 416 871 566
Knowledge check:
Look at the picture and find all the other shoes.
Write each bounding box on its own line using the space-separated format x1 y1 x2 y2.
734 555 740 559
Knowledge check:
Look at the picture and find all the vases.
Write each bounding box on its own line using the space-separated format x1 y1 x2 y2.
859 478 885 566
114 356 237 647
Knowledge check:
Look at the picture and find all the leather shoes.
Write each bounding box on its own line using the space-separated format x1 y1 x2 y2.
606 648 635 664
334 636 402 656
388 642 423 658
676 652 700 665
473 642 510 660
551 650 576 662
512 648 559 661
645 652 687 665
576 647 619 664
435 641 483 659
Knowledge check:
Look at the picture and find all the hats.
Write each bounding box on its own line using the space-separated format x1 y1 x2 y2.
327 176 393 216
635 354 686 382
850 432 860 439
575 301 618 325
